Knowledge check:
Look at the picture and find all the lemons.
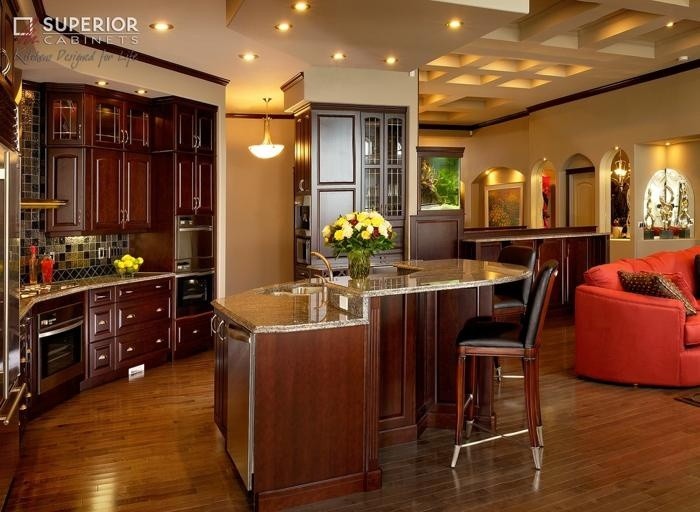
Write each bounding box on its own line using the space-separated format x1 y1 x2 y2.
114 255 144 273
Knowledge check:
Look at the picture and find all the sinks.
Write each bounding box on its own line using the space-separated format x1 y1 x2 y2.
292 286 321 294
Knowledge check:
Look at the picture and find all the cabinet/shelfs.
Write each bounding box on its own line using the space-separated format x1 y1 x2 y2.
378 287 493 447
20 274 175 424
410 216 464 262
462 225 610 329
41 82 154 238
210 304 380 510
154 95 219 363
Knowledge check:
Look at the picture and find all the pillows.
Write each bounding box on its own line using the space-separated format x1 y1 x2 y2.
617 271 700 315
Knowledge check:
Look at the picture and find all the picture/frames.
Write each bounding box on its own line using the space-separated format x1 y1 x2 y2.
484 182 524 228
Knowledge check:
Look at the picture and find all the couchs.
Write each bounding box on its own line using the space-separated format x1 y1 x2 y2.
574 245 700 386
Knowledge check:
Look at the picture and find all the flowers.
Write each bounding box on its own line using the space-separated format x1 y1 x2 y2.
322 210 398 261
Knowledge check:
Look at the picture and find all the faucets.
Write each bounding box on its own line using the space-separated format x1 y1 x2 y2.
311 252 334 282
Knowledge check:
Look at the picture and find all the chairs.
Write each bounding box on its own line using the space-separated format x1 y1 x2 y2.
493 245 537 321
450 259 559 470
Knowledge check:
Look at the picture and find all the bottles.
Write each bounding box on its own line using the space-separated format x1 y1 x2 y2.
40 246 54 284
29 243 37 281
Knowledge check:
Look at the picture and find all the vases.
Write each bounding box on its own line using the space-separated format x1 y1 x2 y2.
348 249 370 280
612 227 623 238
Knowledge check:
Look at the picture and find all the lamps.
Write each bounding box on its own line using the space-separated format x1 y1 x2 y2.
293 102 408 280
247 98 285 160
609 144 632 192
